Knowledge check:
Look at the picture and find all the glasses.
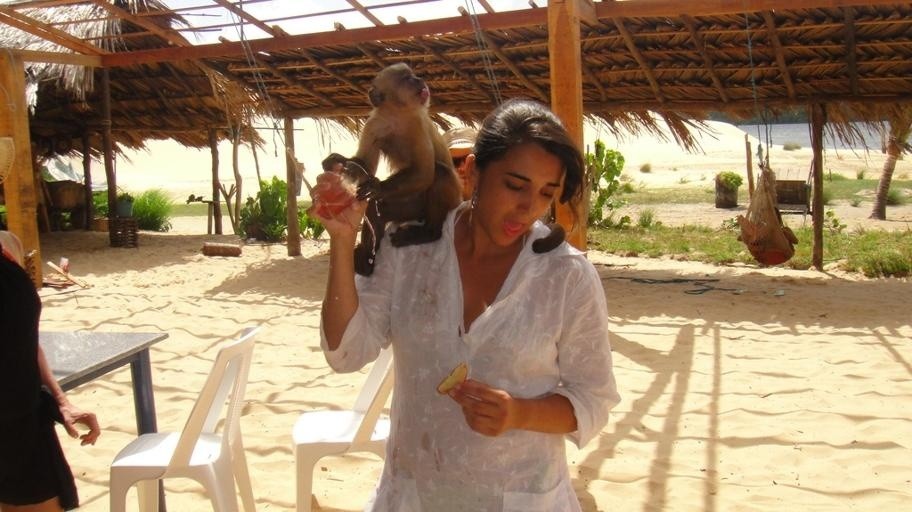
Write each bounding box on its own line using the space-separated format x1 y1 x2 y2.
451 154 469 168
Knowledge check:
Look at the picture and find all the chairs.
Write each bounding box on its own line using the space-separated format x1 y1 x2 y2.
287 344 395 511
105 322 263 512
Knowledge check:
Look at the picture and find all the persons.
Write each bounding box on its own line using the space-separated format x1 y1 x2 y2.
1 229 100 512
736 207 799 267
305 97 621 512
441 125 481 201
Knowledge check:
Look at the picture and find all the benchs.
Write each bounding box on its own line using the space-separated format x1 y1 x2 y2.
771 178 814 226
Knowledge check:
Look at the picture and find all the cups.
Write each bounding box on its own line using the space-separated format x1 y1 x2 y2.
310 161 374 221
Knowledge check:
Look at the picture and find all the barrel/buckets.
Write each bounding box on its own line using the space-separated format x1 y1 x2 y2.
715 174 738 208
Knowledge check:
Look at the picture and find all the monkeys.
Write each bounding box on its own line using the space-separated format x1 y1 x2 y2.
319 56 568 278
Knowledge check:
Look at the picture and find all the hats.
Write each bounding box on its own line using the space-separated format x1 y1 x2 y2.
443 126 479 155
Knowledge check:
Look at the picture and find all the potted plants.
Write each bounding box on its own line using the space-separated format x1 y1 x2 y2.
714 170 743 211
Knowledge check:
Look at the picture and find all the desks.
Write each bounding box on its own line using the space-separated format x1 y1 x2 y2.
34 328 172 512
